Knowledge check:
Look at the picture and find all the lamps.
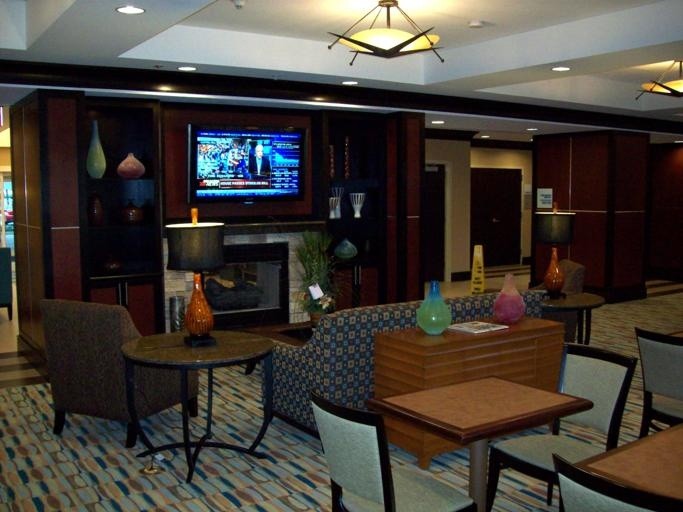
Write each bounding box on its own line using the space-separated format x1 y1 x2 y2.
635 57 683 99
166 220 225 271
328 1 445 65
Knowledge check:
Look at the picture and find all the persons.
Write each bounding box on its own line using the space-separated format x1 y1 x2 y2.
250 144 270 178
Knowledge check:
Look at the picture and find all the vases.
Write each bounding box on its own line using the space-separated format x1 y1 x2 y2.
311 312 323 329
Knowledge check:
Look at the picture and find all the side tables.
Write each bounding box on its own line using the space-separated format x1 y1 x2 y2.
540 291 604 345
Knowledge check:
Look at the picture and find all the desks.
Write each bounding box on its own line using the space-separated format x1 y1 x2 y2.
121 331 275 481
572 422 683 499
367 376 594 512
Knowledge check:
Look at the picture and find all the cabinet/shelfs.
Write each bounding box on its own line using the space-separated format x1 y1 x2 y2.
311 110 424 310
10 90 165 382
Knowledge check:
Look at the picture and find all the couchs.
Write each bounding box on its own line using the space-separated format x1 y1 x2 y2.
532 259 584 342
41 299 198 447
260 290 541 438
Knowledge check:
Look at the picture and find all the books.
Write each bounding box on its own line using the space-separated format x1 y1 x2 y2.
447 320 509 334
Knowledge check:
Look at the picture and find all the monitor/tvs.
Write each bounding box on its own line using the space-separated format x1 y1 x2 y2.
187 122 308 205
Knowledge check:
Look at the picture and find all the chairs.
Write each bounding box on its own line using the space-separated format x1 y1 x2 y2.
311 391 477 512
552 453 683 512
634 326 683 439
486 343 638 512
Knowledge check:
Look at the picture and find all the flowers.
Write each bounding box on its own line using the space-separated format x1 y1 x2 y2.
296 230 337 317
